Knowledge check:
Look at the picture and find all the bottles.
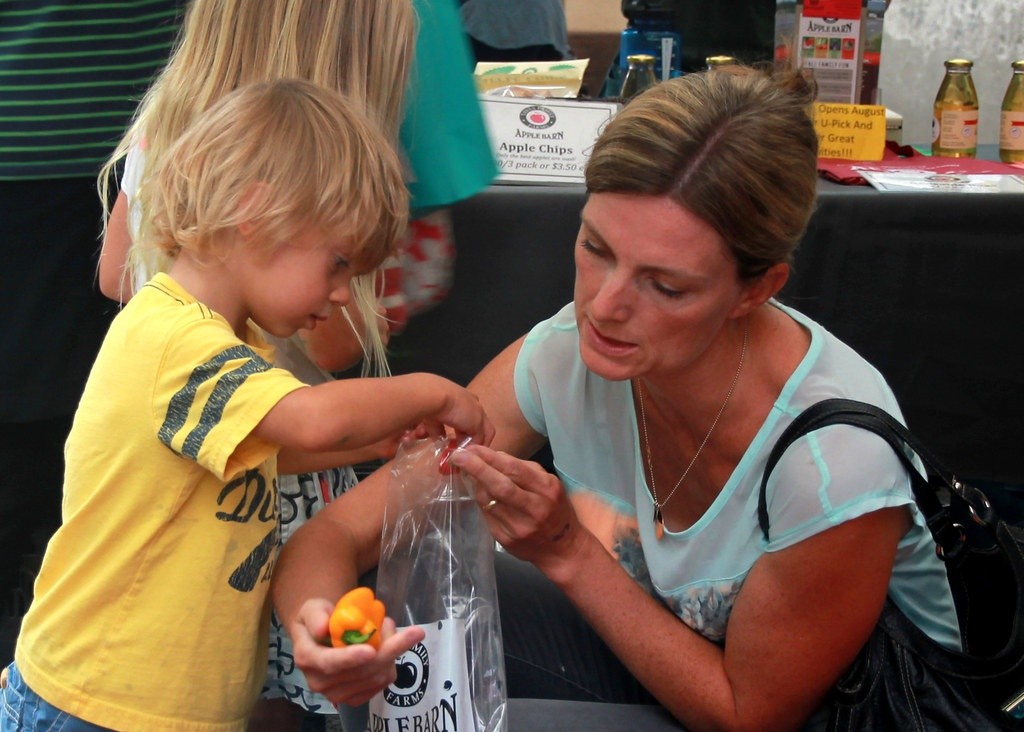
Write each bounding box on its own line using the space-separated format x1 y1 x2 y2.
932 59 979 161
998 60 1024 165
704 54 735 71
618 53 657 104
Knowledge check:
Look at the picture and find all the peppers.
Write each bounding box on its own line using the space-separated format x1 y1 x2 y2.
328 586 385 650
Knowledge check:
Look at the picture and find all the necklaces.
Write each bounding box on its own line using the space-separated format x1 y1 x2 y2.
636 321 748 539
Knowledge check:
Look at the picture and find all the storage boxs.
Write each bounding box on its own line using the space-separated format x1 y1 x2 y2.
774 1 867 104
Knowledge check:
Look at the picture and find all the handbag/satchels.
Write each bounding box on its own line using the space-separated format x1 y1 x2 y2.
759 399 1024 732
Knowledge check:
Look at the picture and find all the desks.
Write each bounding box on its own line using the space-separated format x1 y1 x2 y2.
381 144 1024 482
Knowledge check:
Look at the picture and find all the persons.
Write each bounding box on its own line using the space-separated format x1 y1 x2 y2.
98 0 416 732
0 73 495 732
0 0 189 674
270 64 962 732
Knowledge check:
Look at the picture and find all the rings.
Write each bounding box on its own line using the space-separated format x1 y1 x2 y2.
485 500 497 512
